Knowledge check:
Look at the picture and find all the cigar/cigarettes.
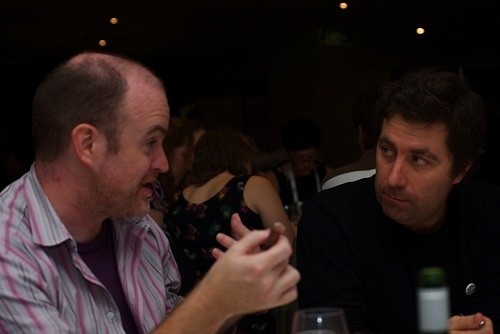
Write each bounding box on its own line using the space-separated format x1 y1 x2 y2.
262 221 285 249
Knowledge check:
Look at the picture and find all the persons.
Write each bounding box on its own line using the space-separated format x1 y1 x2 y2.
147 88 385 334
0 52 300 334
295 63 500 334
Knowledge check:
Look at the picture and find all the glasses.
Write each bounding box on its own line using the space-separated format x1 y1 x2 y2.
291 154 317 163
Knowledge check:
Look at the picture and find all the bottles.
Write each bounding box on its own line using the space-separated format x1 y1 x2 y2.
415 265 451 334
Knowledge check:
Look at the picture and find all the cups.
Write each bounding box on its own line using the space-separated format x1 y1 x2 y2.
289 306 345 334
